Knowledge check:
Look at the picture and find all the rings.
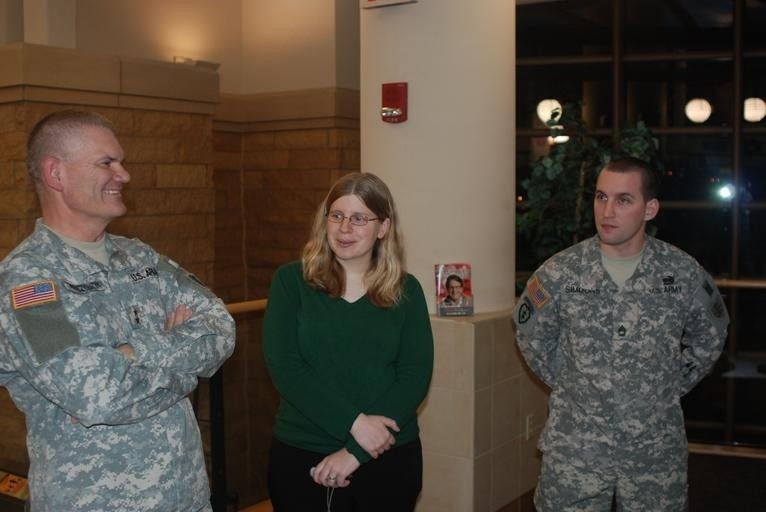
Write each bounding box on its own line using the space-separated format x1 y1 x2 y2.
328 476 337 480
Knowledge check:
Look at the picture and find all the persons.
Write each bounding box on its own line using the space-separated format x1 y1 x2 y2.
513 159 730 511
439 274 472 305
264 172 433 512
0 110 236 512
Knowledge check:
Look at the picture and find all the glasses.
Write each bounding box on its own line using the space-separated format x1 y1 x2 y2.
325 210 383 226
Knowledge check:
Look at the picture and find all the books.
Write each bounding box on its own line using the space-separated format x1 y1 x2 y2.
434 263 473 318
